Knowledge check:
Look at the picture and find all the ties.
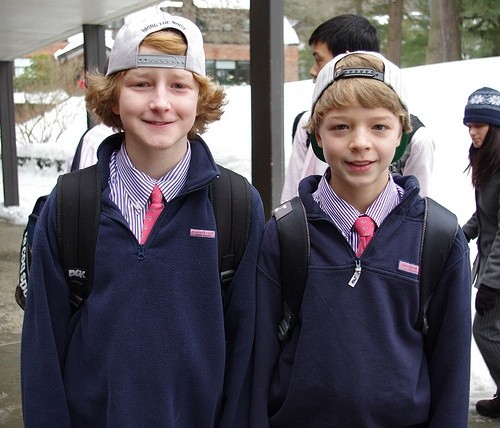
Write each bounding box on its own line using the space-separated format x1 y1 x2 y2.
141 184 165 246
354 216 376 258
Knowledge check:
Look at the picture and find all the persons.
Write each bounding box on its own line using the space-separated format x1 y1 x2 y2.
280 13 434 210
18 16 266 428
463 85 500 420
251 49 476 428
69 120 125 171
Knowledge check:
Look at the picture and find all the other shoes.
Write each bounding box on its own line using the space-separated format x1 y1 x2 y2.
476 396 500 419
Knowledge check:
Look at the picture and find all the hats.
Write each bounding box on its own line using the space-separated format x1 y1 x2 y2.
105 13 206 78
311 50 409 164
463 87 500 126
